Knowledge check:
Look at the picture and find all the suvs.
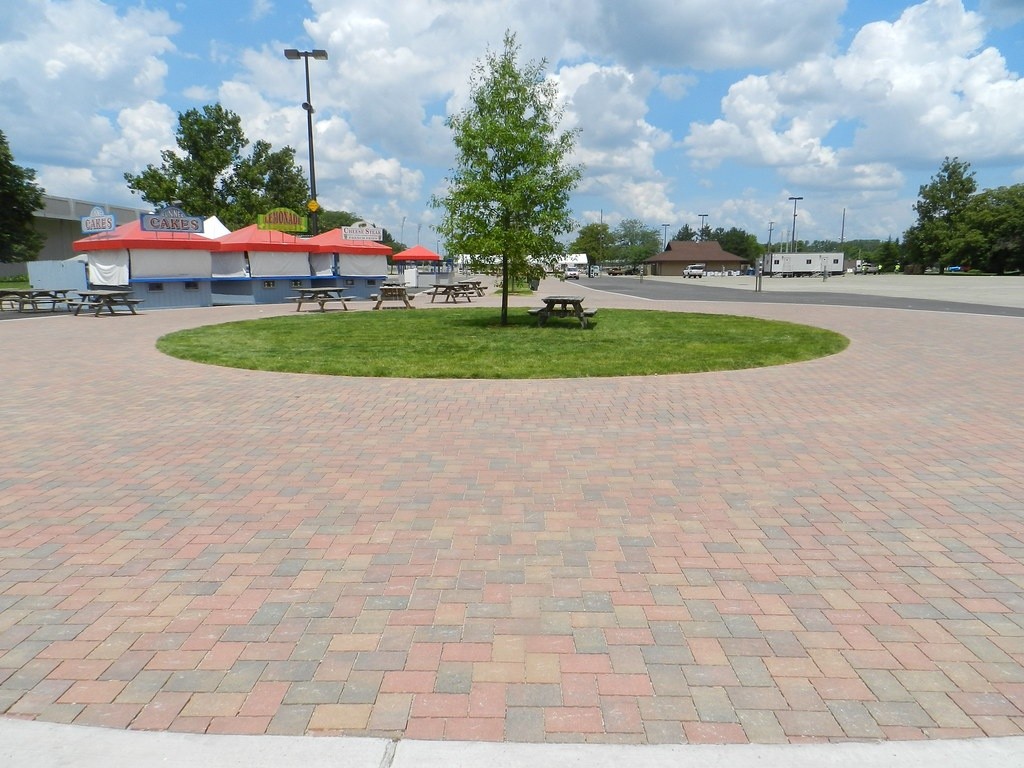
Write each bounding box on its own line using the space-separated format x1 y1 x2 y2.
565 266 579 280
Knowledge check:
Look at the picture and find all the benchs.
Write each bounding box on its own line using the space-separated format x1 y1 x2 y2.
370 293 378 300
106 299 144 304
0 297 80 304
317 296 356 303
456 290 476 296
479 286 488 289
583 307 598 317
409 293 415 299
67 302 102 307
528 307 547 317
424 290 443 294
286 296 314 301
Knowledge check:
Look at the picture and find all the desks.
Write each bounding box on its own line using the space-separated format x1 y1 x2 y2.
0 288 19 311
291 287 348 313
74 290 136 317
7 290 51 314
36 289 76 313
428 284 472 303
371 287 411 310
538 296 590 328
382 282 406 287
456 281 485 297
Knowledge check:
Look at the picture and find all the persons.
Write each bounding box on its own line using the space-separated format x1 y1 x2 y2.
721 265 726 276
894 263 900 271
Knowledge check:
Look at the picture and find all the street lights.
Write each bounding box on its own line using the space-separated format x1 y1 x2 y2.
662 224 671 251
284 48 328 236
698 214 708 228
788 197 804 254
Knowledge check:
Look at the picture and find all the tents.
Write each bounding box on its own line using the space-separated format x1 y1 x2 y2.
390 244 440 275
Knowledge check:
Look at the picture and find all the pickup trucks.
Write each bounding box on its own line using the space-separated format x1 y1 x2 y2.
682 263 703 279
861 263 877 276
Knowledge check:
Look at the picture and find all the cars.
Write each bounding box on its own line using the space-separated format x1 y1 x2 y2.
948 265 964 272
